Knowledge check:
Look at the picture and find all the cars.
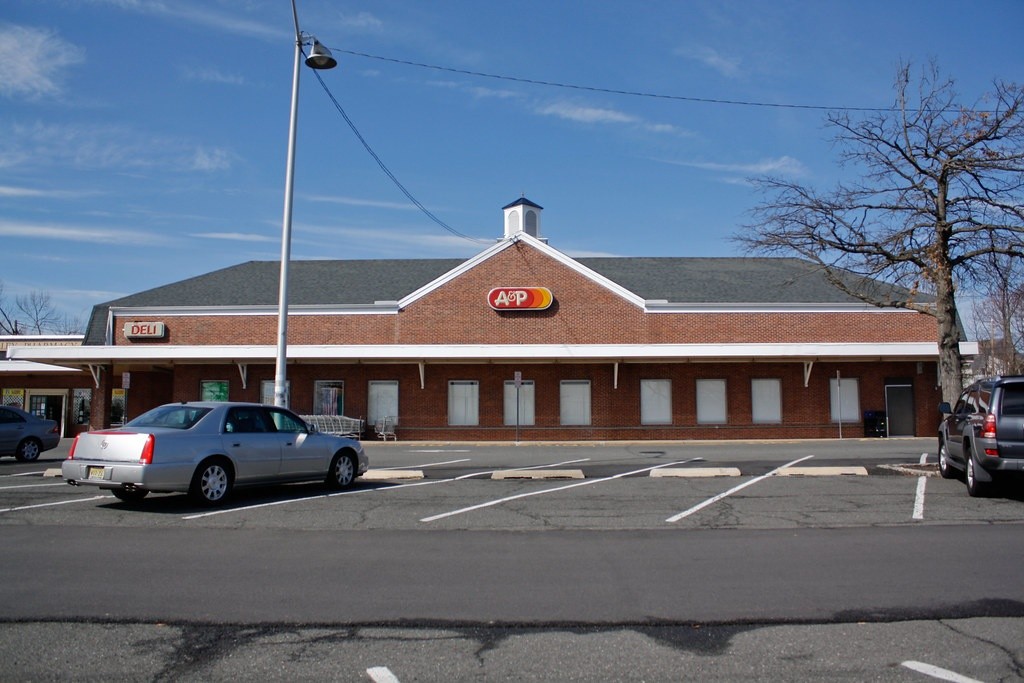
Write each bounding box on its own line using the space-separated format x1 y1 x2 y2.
61 401 370 510
0 406 61 463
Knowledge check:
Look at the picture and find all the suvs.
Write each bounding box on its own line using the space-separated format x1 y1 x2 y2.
936 374 1024 497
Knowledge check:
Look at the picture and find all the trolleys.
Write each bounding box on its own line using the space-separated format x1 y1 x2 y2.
374 415 399 442
283 414 366 441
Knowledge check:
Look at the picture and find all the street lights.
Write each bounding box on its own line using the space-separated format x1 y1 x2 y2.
271 31 341 432
